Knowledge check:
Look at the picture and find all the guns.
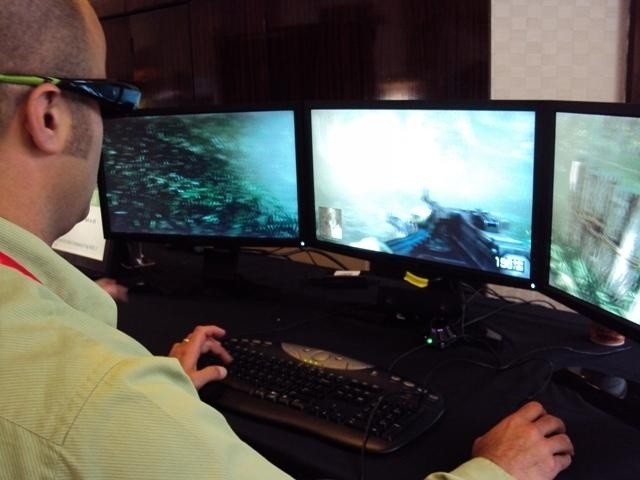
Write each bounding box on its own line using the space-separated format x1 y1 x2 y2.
384 188 500 272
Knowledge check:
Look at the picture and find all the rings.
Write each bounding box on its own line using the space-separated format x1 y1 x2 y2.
183 338 189 343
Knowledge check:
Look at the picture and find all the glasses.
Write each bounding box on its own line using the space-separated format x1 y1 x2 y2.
0 74 143 119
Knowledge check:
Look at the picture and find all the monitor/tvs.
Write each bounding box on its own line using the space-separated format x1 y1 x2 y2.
94 105 305 303
538 101 640 346
52 188 113 274
307 101 539 325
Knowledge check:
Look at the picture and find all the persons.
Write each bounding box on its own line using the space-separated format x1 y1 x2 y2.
95 277 129 302
0 0 574 480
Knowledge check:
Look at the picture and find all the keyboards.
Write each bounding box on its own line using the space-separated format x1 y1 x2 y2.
196 333 443 455
